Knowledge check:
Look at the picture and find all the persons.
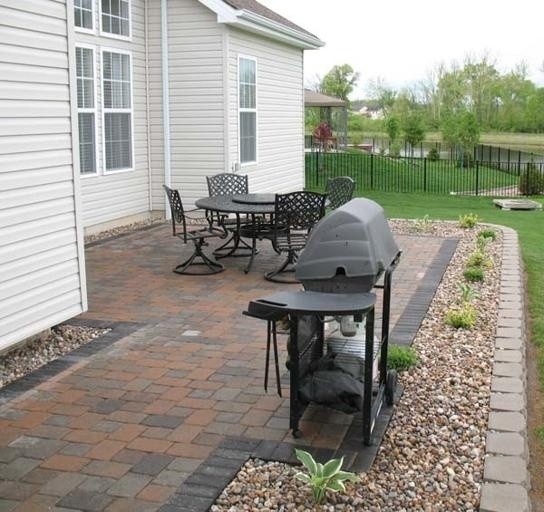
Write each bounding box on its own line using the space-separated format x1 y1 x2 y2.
312 121 331 151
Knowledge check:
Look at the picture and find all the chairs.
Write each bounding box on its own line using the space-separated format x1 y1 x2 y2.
162 184 228 276
205 172 265 257
263 190 328 284
324 176 356 210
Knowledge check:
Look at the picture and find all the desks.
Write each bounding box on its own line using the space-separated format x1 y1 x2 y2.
194 192 332 275
